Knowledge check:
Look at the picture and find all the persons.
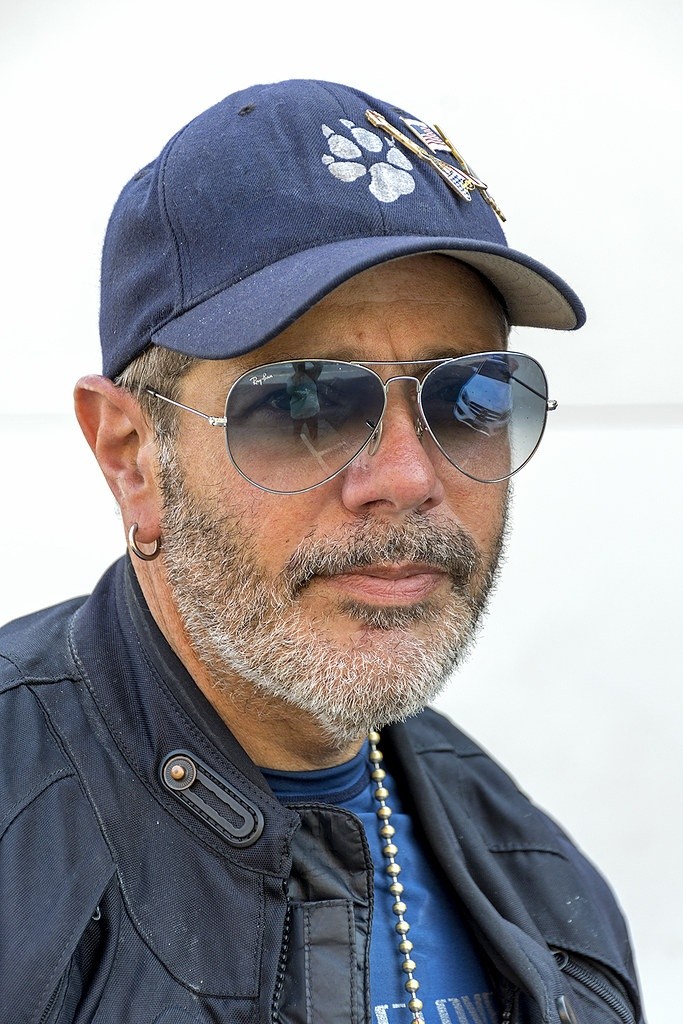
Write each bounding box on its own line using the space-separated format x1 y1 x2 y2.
0 77 647 1024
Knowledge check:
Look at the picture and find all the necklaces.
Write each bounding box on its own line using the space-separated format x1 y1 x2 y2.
371 725 425 1023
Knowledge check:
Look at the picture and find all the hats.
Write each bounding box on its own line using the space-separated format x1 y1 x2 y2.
100 80 586 375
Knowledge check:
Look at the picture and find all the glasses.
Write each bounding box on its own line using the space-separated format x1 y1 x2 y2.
130 352 558 495
